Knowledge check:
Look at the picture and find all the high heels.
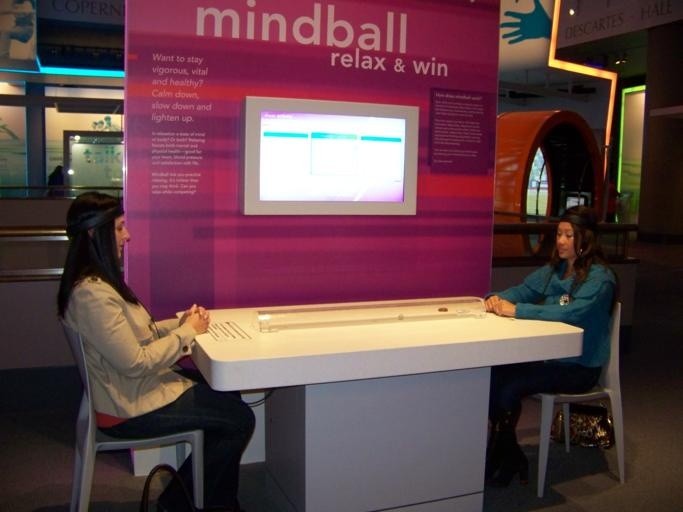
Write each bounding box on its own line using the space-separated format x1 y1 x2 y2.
486 440 529 486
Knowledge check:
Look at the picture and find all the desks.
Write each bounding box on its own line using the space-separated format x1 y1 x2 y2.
173 294 587 512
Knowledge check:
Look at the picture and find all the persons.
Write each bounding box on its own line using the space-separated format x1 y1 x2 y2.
47 166 65 197
485 205 620 487
56 192 256 512
606 173 622 223
0 0 33 42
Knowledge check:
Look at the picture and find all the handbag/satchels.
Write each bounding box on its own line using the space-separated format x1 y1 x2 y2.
551 404 614 448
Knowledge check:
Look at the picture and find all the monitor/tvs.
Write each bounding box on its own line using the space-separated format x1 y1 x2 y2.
243 96 420 216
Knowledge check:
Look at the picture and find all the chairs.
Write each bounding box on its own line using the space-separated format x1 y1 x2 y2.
526 302 627 498
61 318 206 512
